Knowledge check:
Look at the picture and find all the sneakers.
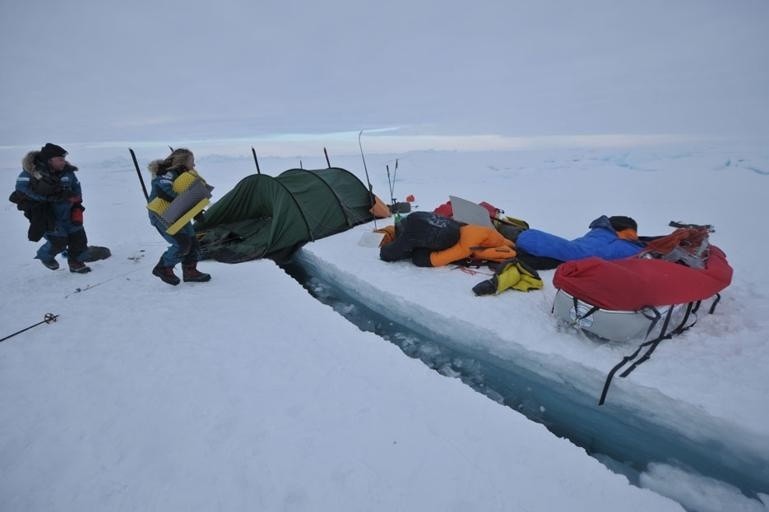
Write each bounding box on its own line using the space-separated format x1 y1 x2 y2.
42 254 59 271
183 268 211 283
152 263 180 286
70 263 92 274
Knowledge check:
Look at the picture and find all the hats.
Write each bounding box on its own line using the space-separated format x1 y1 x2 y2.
41 142 69 159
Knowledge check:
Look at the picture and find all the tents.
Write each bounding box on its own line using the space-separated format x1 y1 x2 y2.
191 165 392 266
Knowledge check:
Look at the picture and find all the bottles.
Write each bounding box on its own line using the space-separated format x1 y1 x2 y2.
394 211 402 233
60 177 70 193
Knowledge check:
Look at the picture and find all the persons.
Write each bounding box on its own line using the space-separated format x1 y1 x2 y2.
516 215 642 269
15 143 92 274
145 147 213 287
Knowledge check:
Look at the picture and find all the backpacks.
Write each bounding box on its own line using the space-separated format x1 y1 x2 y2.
394 210 461 251
